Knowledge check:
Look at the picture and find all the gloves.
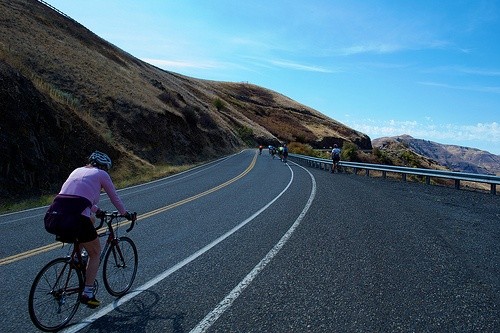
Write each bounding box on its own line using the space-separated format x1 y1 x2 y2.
95 210 106 218
125 212 134 221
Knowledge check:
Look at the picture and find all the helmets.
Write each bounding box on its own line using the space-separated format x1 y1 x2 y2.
88 150 112 174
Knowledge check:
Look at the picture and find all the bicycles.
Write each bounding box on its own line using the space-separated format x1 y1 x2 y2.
28 209 138 332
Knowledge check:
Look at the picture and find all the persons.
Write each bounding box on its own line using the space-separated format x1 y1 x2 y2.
327 144 341 173
268 144 288 163
45 151 134 305
259 145 263 154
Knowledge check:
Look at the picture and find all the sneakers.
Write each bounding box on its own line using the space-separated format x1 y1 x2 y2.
79 294 100 306
73 260 88 270
